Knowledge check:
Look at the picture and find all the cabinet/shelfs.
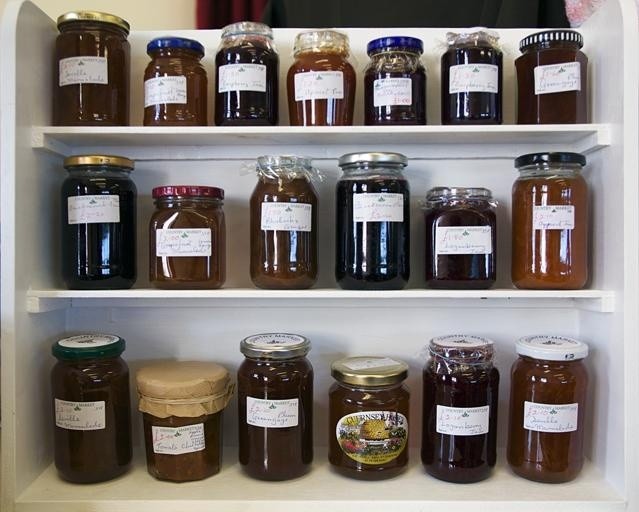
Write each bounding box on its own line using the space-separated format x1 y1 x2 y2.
0 0 638 511
